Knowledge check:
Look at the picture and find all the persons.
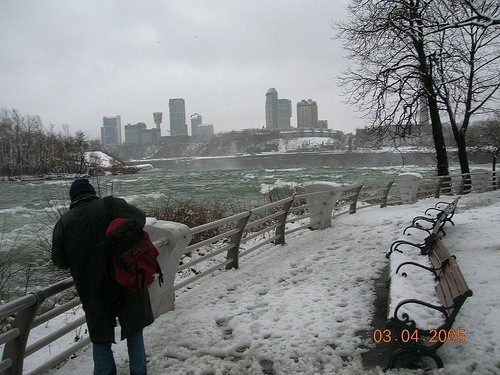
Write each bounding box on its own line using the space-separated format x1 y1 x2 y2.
51 179 155 375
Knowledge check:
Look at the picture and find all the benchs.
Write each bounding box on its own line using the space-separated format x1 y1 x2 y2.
385 195 461 259
383 235 473 374
386 233 452 289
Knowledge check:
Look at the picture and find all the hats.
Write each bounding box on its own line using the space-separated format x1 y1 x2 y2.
70 179 97 202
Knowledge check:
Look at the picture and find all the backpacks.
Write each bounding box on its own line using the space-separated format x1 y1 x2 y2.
103 194 163 297
